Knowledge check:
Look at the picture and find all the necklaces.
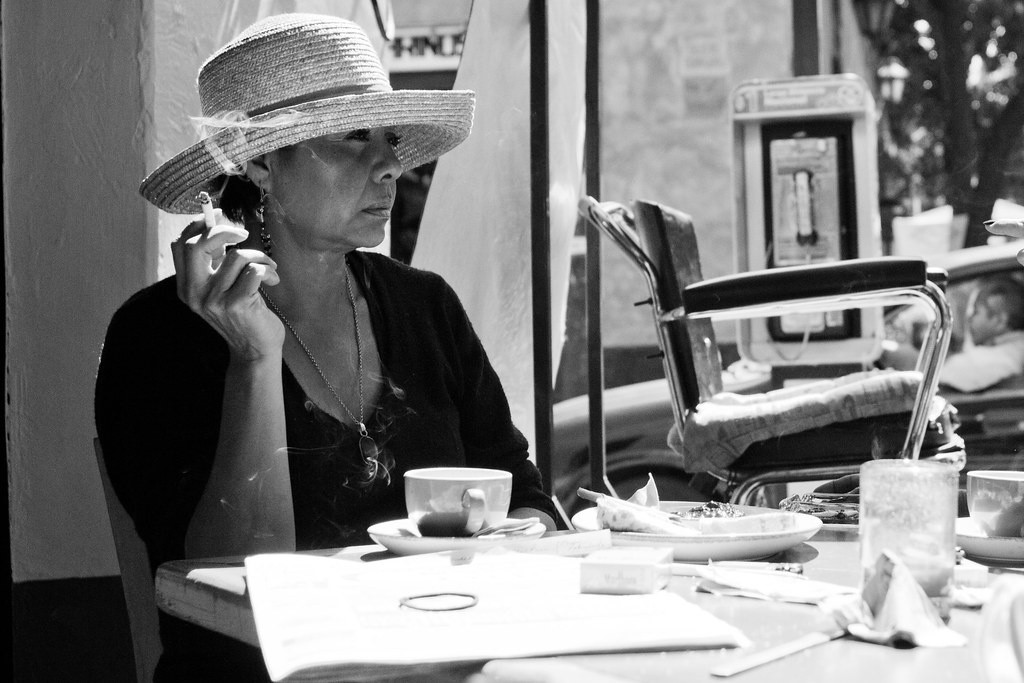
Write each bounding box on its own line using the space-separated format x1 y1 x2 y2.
259 266 379 466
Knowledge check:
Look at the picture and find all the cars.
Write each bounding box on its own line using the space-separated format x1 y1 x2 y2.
547 238 1024 521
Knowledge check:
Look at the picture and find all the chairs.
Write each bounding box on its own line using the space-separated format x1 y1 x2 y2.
569 194 963 503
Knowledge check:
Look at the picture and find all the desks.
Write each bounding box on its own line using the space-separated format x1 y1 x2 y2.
151 520 1024 683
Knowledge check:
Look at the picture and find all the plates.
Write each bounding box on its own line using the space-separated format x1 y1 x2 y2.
367 518 546 555
823 524 859 532
954 517 1024 561
571 501 823 562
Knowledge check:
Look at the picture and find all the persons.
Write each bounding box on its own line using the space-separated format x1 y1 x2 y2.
93 12 559 683
882 277 1024 393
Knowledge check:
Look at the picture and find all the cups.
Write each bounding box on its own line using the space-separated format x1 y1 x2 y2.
403 467 513 537
859 460 959 622
966 471 1024 537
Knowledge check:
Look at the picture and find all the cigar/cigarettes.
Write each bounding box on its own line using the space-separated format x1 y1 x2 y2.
197 191 216 230
